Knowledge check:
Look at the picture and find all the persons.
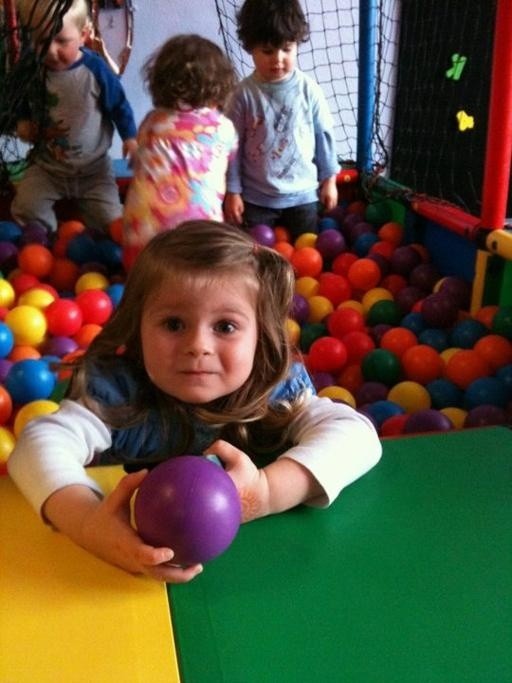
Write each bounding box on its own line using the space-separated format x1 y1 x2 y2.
2 1 143 239
219 1 345 244
118 30 244 269
11 218 385 584
